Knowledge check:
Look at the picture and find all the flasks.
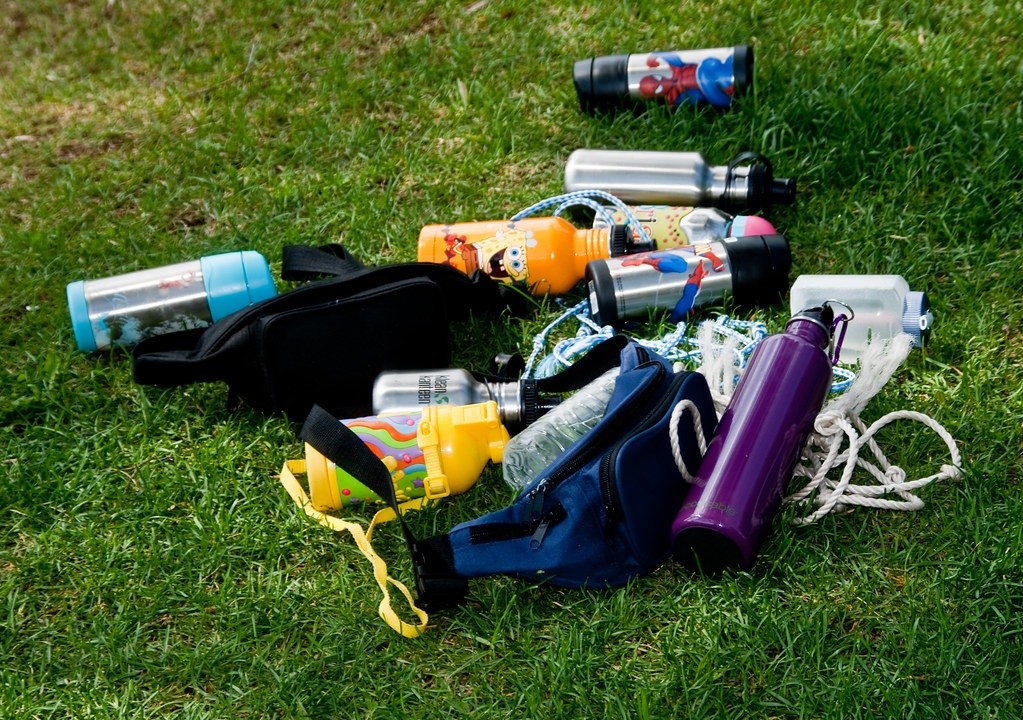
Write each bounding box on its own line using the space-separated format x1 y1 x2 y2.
372 366 566 425
562 148 796 210
572 44 754 116
670 298 854 574
585 234 790 323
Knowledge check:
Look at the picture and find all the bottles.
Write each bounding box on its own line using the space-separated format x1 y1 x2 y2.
789 273 932 363
501 366 620 490
589 203 777 248
304 401 511 514
66 251 276 354
417 216 656 296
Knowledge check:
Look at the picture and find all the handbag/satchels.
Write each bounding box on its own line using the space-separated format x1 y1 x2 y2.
406 340 719 614
127 239 501 432
